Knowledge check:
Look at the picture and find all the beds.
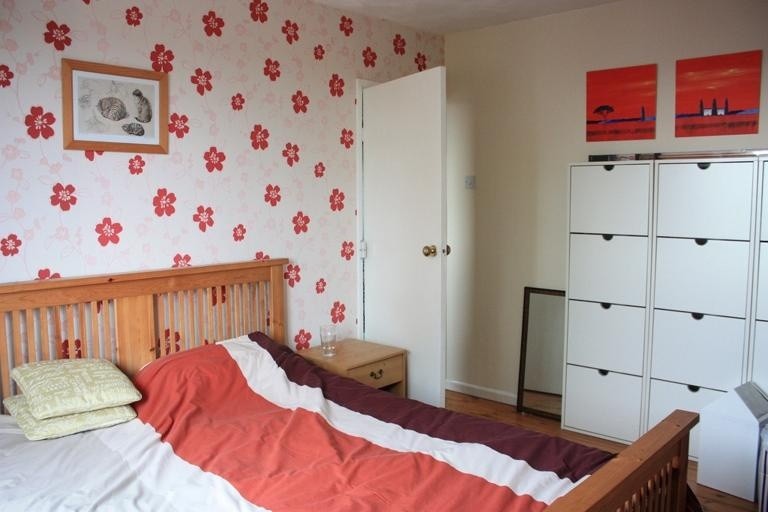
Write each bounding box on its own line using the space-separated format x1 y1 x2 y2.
0 258 699 511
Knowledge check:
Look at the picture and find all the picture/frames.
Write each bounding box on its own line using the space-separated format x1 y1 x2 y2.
61 59 170 153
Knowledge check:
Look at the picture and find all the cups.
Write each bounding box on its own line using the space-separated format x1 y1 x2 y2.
320 324 337 357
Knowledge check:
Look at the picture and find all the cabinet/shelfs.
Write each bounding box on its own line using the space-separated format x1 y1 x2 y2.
560 153 768 468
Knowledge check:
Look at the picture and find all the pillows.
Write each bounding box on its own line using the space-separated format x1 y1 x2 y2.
3 333 143 441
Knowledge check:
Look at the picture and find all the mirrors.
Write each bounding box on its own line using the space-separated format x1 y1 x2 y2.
515 286 565 421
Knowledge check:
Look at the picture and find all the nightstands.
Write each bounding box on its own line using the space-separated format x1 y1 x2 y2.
297 336 409 403
